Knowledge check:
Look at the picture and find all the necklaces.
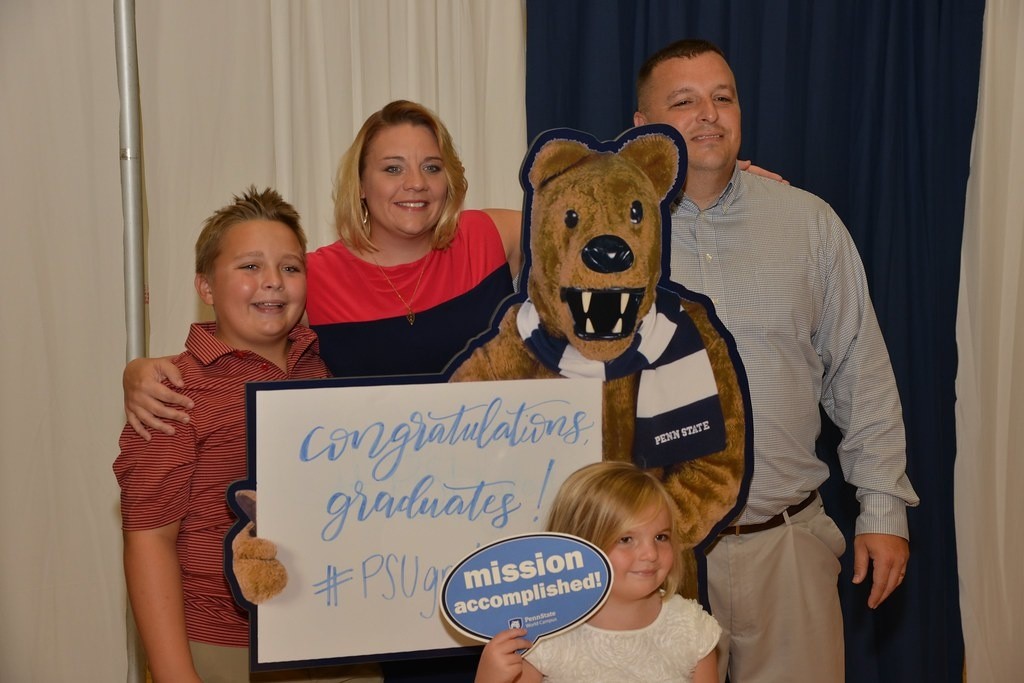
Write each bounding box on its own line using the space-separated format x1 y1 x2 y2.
371 242 431 325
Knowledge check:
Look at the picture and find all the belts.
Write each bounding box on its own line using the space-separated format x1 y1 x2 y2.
721 490 816 535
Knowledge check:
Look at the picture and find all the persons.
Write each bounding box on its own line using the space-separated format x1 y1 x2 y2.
473 461 723 683
123 100 791 683
112 183 328 683
633 40 920 683
